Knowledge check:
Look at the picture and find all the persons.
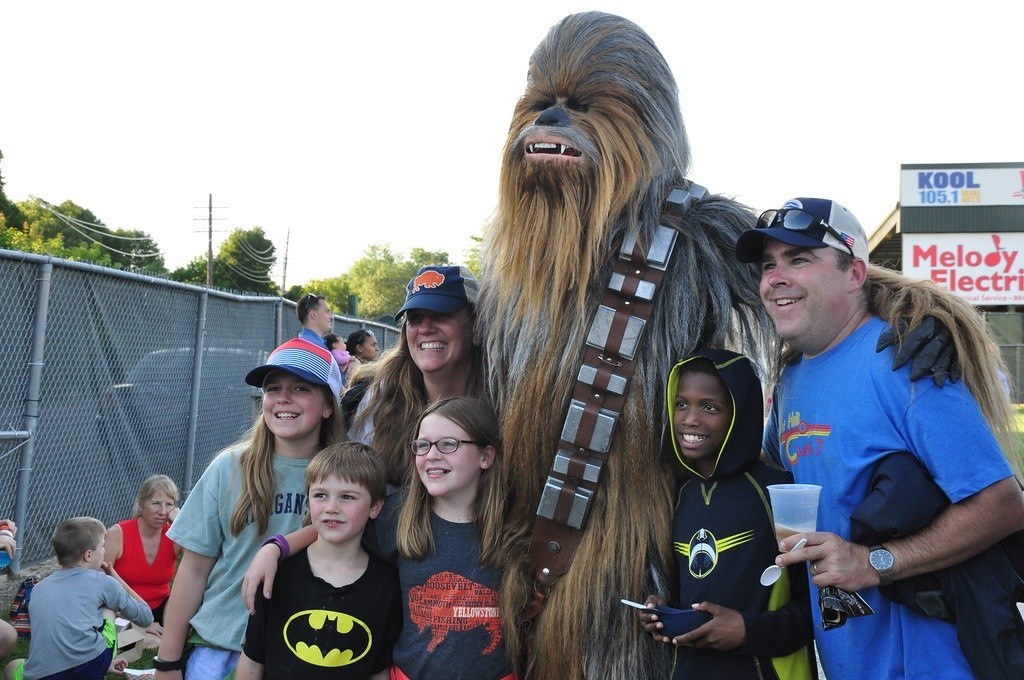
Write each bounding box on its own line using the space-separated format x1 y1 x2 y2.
235 441 401 680
241 397 518 680
347 266 480 493
296 293 346 385
324 334 355 387
638 348 813 680
347 331 380 385
4 517 155 680
103 475 195 637
736 197 1024 680
152 338 342 680
0 519 17 658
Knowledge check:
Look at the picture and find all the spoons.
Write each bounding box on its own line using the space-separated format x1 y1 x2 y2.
759 540 808 586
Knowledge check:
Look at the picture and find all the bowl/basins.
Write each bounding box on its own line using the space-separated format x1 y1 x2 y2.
650 604 708 637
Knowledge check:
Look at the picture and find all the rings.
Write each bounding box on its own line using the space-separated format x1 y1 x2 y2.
814 563 821 575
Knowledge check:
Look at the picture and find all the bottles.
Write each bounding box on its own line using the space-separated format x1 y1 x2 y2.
0 525 16 569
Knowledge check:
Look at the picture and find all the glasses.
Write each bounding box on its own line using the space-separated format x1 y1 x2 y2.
358 330 374 344
410 438 486 456
756 209 856 259
306 293 316 315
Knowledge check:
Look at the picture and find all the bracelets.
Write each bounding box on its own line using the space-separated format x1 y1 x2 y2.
151 656 185 672
258 534 290 561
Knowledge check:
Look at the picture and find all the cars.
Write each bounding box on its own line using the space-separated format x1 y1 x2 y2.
94 346 273 470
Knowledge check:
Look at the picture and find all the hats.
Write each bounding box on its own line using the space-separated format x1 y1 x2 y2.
246 339 342 399
395 267 479 322
736 198 869 266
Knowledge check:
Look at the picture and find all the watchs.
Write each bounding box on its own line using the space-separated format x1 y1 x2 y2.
868 544 895 588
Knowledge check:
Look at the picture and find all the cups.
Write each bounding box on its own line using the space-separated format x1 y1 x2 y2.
767 484 824 553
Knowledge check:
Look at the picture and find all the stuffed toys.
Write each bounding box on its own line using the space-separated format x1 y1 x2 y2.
230 10 1024 680
